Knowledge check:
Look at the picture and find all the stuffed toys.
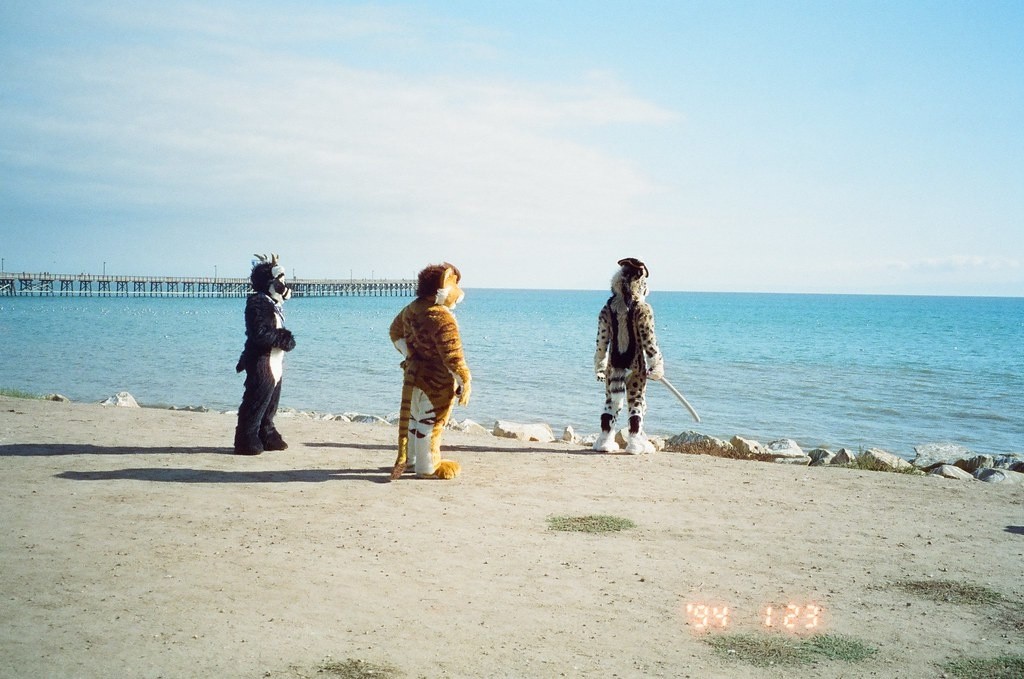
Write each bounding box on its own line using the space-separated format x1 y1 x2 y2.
234 252 296 456
593 257 665 454
389 262 471 480
